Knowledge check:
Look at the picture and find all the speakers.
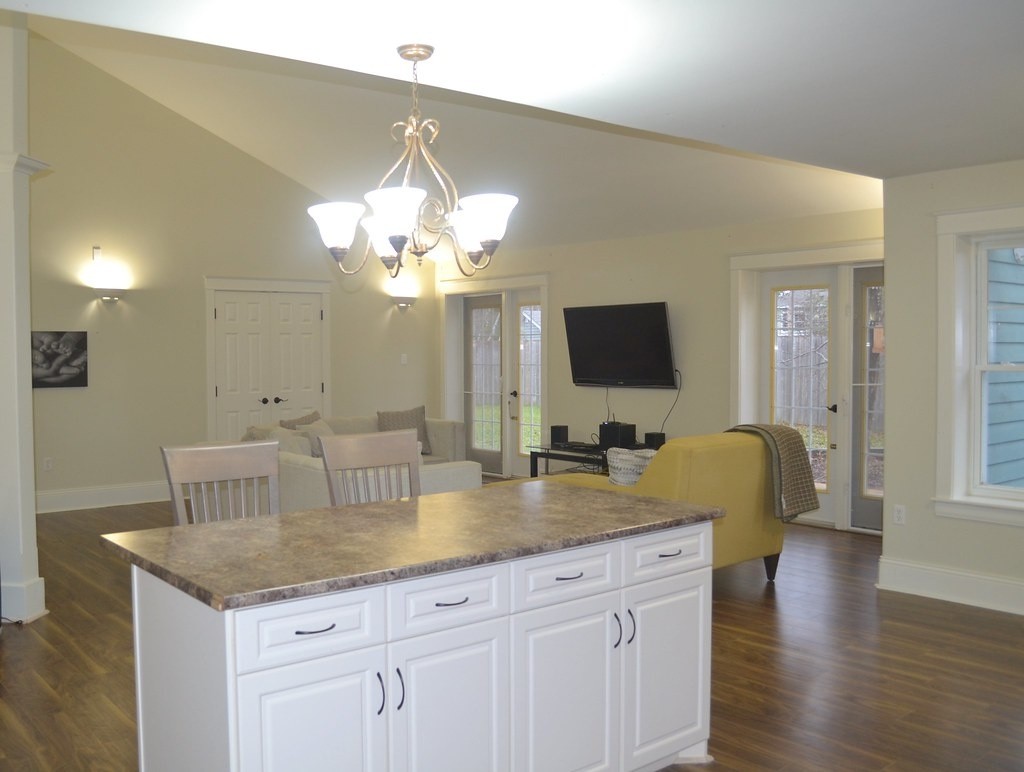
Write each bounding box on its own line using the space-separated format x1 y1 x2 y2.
645 432 665 450
551 425 568 450
599 423 636 450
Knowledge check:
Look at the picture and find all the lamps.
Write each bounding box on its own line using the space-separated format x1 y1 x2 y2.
307 45 519 277
391 297 417 312
93 288 129 303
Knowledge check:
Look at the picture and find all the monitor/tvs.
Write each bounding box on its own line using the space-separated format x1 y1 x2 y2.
564 301 678 390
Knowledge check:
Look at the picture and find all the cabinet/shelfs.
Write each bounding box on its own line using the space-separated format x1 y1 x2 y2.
525 442 645 478
511 520 714 772
130 563 511 772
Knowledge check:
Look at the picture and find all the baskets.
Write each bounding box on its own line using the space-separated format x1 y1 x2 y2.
606 447 657 487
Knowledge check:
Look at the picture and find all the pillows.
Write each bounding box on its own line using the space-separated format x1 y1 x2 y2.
246 426 277 442
279 410 321 431
296 419 335 458
269 428 311 455
376 406 432 454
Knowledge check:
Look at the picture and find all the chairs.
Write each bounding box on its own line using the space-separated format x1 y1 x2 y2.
317 428 421 506
483 432 785 580
159 441 280 526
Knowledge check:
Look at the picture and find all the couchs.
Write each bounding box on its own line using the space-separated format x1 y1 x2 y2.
193 415 482 522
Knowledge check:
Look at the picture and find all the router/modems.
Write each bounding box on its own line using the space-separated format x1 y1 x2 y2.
603 413 621 425
